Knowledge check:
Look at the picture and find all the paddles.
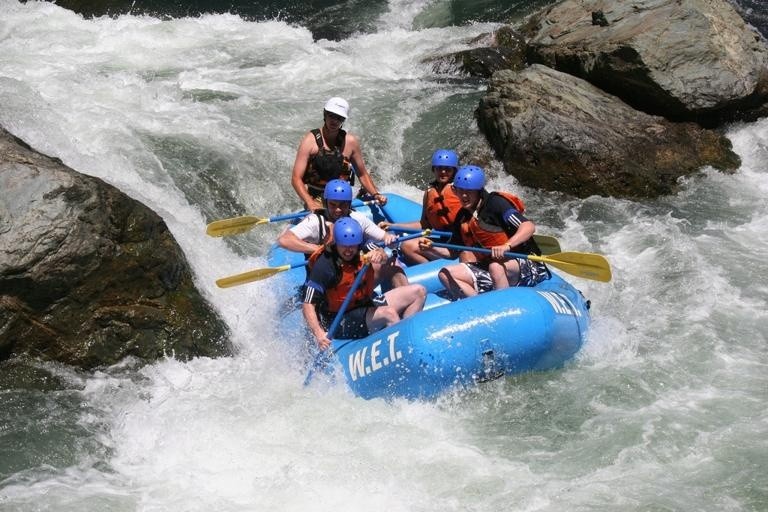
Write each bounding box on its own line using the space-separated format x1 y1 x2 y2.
428 241 612 282
386 226 560 256
207 200 377 237
216 229 431 288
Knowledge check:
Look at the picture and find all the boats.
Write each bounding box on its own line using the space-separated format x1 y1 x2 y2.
264 193 593 402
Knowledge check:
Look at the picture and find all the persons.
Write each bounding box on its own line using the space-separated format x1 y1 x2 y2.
278 178 394 281
376 149 462 266
418 164 536 300
289 95 388 214
303 216 427 354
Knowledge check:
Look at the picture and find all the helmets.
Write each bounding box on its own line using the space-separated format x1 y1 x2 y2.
453 165 484 192
323 179 351 204
322 98 350 121
333 216 362 246
432 150 457 170
